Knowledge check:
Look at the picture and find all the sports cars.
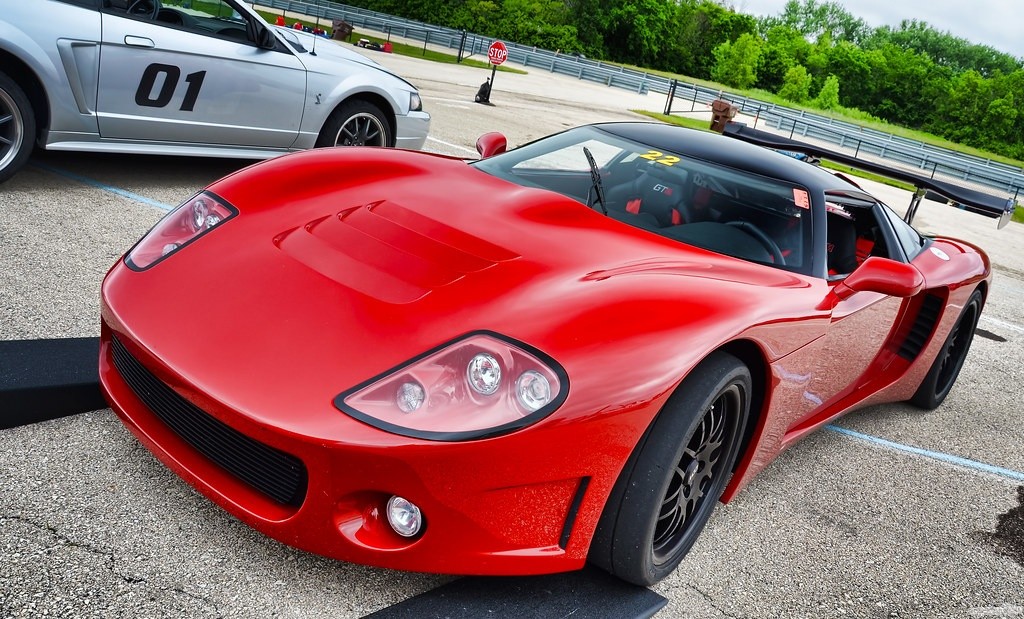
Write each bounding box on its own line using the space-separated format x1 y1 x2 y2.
0 1 434 186
95 120 993 590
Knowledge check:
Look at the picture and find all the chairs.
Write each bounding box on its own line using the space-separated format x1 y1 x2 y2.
608 165 687 228
801 209 856 271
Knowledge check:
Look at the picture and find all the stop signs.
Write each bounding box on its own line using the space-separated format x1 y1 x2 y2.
487 42 508 65
